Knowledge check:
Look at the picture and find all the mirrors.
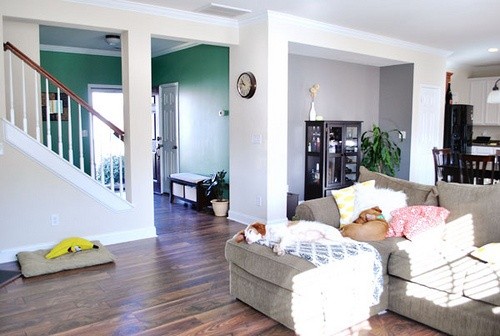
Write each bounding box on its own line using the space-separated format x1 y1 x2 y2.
41 91 68 121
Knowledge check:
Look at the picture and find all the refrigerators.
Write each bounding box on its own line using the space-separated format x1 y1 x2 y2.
443 105 474 185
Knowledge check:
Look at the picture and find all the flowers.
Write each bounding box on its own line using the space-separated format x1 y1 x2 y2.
310 84 320 101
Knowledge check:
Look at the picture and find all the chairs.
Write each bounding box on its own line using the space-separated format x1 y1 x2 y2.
432 147 500 184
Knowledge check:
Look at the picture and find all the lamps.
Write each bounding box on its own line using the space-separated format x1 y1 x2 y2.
487 78 500 103
104 34 120 46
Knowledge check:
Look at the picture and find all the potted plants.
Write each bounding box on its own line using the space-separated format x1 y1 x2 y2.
209 169 229 218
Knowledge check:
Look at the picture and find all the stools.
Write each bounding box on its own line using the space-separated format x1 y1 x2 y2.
225 219 390 336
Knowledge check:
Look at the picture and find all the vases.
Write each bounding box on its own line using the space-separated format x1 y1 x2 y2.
309 102 316 121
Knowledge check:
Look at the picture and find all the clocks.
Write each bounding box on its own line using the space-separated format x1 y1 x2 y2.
236 71 257 99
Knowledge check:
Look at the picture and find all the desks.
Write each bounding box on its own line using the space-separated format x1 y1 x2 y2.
437 162 500 184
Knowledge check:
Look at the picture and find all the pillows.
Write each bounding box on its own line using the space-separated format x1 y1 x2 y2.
386 205 449 240
351 179 408 219
20 239 116 280
331 179 376 228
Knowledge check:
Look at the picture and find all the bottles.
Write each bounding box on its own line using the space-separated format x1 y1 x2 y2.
314 163 320 182
316 138 320 152
307 142 312 152
311 169 315 182
311 136 316 152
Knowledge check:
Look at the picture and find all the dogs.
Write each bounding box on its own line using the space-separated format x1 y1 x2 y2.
340 206 388 241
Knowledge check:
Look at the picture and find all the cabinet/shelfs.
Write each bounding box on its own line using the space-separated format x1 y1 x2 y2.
445 104 474 183
167 177 213 210
467 76 500 126
304 119 363 201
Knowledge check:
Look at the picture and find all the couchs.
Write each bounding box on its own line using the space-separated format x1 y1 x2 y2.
292 166 500 336
